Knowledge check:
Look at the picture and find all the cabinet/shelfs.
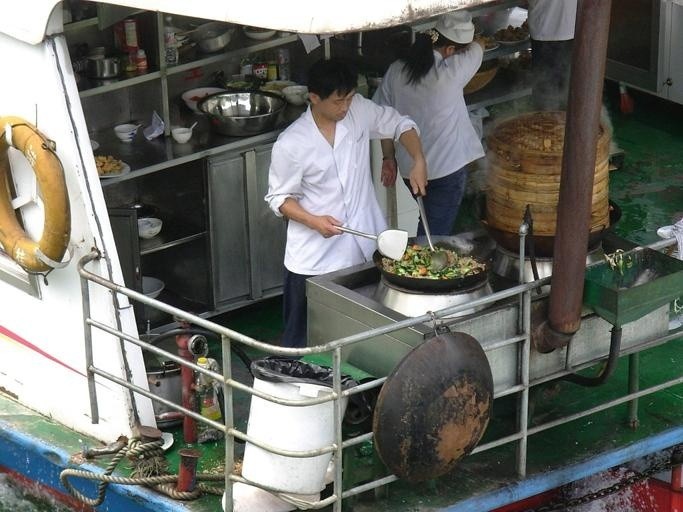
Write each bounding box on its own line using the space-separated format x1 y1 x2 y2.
64 1 329 326
331 3 532 200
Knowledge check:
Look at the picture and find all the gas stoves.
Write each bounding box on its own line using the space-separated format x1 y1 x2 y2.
334 221 634 319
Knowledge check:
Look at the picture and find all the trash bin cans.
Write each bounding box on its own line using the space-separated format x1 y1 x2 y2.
241 356 360 495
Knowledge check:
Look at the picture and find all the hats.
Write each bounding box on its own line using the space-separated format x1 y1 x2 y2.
433 9 475 45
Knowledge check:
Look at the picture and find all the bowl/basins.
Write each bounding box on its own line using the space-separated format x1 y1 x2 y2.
113 124 141 142
201 90 290 137
243 26 278 40
190 23 233 52
171 126 192 145
143 274 164 301
464 60 501 96
181 86 227 115
283 84 309 105
136 218 164 240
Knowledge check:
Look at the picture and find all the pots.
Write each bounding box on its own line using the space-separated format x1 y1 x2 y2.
370 234 500 293
139 332 200 427
370 325 494 484
471 193 620 256
79 51 133 79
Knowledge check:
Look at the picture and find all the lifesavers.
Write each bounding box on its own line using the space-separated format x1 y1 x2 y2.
0 117 70 271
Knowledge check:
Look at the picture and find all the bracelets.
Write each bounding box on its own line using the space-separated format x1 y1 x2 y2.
382 156 396 160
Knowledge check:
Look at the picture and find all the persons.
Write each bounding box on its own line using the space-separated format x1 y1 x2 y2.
378 9 486 236
263 63 428 360
527 0 577 111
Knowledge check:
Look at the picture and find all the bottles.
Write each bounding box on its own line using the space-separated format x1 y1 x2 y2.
165 33 178 66
240 49 292 80
116 17 152 70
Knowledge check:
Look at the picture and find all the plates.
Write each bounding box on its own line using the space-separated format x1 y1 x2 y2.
656 224 676 240
473 23 532 53
260 80 298 95
90 155 130 176
163 26 188 40
89 138 100 152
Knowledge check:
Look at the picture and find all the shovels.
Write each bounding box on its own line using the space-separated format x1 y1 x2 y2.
416 188 449 272
332 225 408 262
